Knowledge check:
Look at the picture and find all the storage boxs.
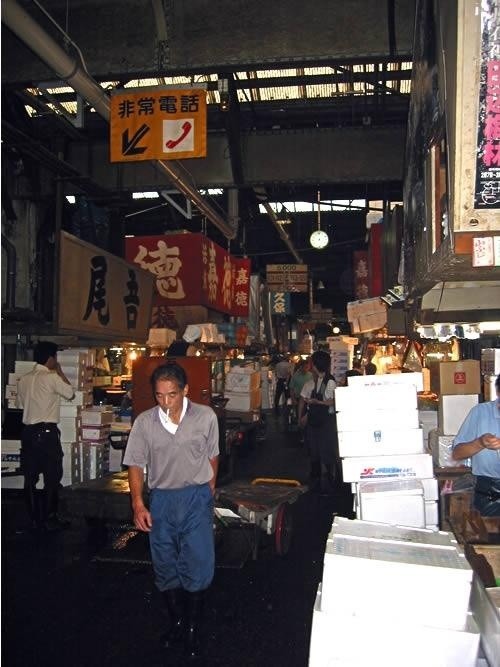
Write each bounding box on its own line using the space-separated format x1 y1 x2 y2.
224 362 281 413
326 336 359 385
307 516 500 667
333 348 499 534
2 348 124 487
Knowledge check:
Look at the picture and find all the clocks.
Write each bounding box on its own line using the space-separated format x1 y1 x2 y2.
308 230 329 250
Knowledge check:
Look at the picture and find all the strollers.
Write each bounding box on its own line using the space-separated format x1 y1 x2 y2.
285 396 310 434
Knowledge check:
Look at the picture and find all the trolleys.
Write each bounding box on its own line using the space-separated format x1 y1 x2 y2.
205 473 311 555
211 397 244 451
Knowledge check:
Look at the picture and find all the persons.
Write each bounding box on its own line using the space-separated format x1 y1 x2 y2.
14 341 76 532
272 351 376 480
450 374 500 517
121 363 220 661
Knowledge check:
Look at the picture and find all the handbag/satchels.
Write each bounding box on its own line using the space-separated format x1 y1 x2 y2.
31 429 64 464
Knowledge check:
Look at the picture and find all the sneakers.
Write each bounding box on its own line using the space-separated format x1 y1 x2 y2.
29 514 69 531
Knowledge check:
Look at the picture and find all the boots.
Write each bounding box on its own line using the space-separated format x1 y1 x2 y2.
304 461 324 486
158 589 187 647
185 591 206 662
325 462 342 488
